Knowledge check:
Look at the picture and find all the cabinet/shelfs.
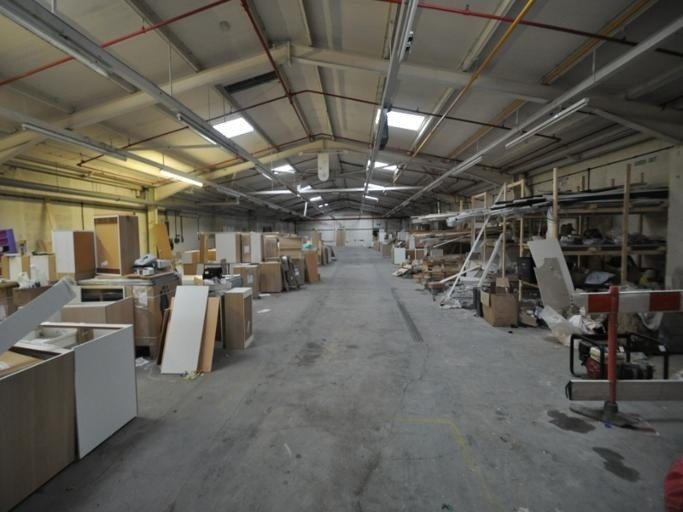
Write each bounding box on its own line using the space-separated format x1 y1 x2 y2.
372 230 468 286
469 162 668 327
181 229 323 350
